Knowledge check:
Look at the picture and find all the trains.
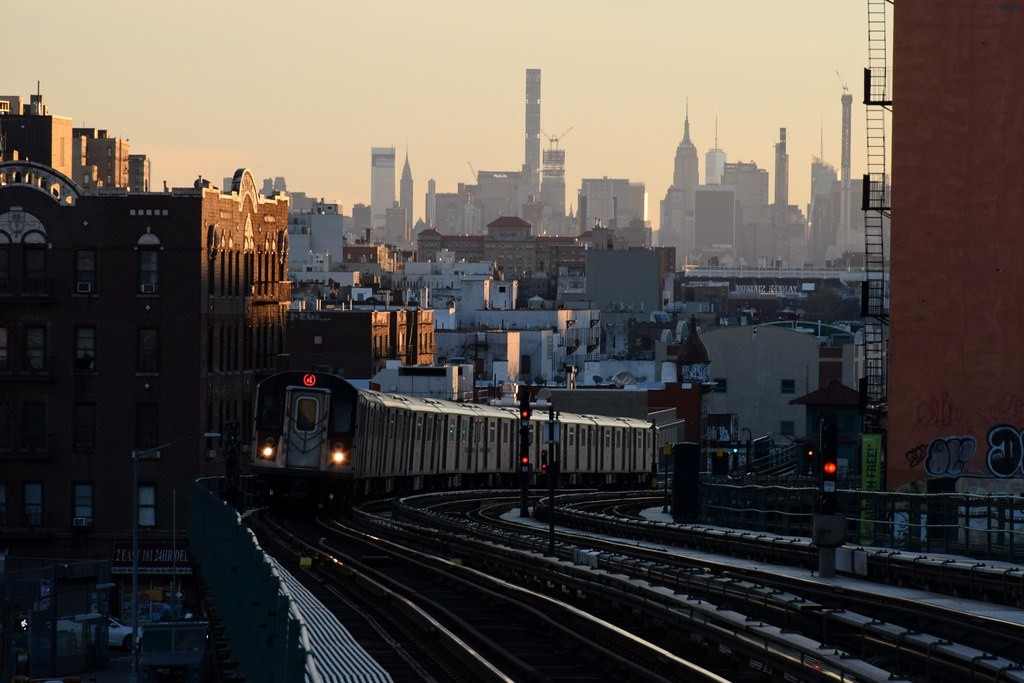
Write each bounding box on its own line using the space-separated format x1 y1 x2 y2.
248 368 661 519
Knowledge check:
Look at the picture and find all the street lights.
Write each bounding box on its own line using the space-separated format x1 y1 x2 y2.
129 430 222 678
750 319 868 405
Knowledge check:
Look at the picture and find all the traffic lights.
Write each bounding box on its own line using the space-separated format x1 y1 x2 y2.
516 382 531 473
819 411 837 495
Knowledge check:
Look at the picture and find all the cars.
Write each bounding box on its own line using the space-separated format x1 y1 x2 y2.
45 612 144 652
